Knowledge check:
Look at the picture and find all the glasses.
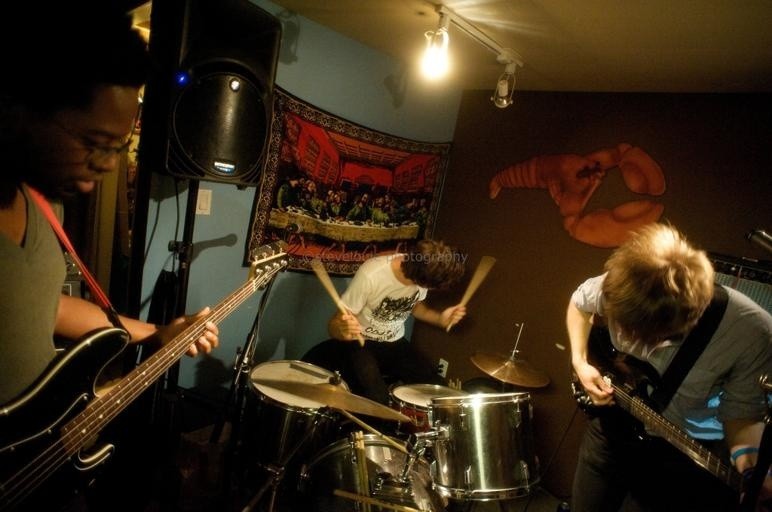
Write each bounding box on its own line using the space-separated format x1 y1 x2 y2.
54 117 132 161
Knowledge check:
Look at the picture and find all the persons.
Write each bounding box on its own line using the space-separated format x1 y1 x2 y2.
272 174 429 231
566 222 771 511
1 13 223 511
325 237 471 404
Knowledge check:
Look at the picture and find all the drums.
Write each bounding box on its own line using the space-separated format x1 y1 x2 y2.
305 433 442 511
385 383 471 442
227 358 350 477
426 390 542 503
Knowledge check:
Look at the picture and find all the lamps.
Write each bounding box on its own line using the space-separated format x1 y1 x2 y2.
489 65 519 108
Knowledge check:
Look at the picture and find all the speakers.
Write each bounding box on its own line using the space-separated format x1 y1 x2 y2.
136 0 282 191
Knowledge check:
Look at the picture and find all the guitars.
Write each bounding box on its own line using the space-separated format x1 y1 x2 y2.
569 323 770 511
0 248 292 510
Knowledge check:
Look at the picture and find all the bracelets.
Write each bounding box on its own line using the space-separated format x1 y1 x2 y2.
729 447 759 466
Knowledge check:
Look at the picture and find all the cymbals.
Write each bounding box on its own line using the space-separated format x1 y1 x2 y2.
469 350 551 389
248 378 411 423
